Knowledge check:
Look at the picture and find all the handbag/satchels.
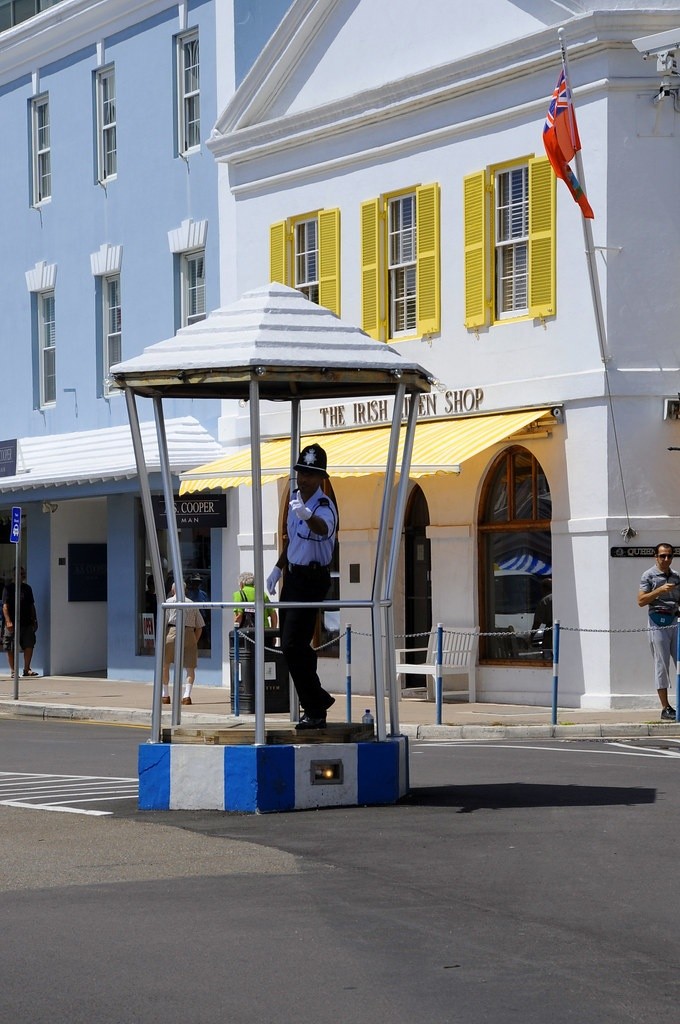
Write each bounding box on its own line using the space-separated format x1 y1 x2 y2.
239 590 256 627
649 607 678 626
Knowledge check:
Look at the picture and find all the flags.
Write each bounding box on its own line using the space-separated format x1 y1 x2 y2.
544 67 595 219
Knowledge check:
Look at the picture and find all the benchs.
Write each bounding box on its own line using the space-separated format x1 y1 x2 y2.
396 624 480 703
495 624 543 660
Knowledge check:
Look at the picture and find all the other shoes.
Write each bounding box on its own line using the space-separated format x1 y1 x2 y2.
162 697 170 704
661 705 676 719
182 697 191 705
299 689 335 723
295 717 327 729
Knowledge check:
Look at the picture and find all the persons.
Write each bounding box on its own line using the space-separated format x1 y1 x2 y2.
637 544 680 719
266 443 337 730
163 581 203 706
234 573 277 651
1 567 39 678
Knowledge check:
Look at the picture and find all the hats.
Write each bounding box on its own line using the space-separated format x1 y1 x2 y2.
293 443 329 479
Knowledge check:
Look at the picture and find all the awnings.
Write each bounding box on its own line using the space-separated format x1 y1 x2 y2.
177 408 552 497
495 553 552 577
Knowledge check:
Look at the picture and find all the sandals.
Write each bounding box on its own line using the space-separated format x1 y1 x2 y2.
22 668 39 678
11 671 20 678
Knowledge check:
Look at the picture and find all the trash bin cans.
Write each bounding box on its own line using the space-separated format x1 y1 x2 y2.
229 628 290 714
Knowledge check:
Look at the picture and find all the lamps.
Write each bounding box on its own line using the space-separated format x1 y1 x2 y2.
42 502 58 513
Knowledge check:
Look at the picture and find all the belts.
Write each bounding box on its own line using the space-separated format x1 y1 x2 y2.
168 625 195 631
287 562 330 574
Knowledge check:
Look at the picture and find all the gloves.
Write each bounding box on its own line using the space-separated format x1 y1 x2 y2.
289 490 312 520
268 566 282 595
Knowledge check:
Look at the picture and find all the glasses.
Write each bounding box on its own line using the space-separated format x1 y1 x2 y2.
21 572 26 576
657 554 673 559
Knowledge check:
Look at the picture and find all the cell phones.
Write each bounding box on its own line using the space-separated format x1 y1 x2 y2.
674 582 680 586
